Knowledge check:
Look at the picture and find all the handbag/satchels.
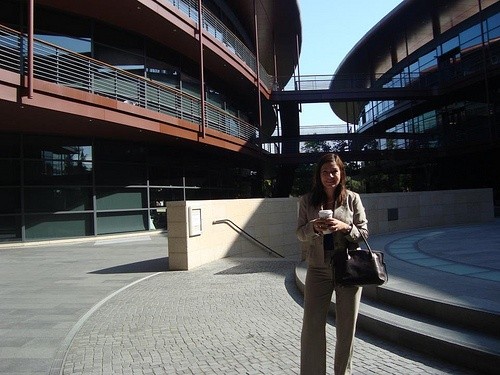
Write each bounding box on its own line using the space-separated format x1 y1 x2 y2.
329 228 389 288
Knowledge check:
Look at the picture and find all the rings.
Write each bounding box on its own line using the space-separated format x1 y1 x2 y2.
319 225 322 228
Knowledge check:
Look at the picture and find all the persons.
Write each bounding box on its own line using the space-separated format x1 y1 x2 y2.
297 152 370 375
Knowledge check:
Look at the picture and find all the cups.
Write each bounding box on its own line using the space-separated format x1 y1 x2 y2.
320 209 334 234
